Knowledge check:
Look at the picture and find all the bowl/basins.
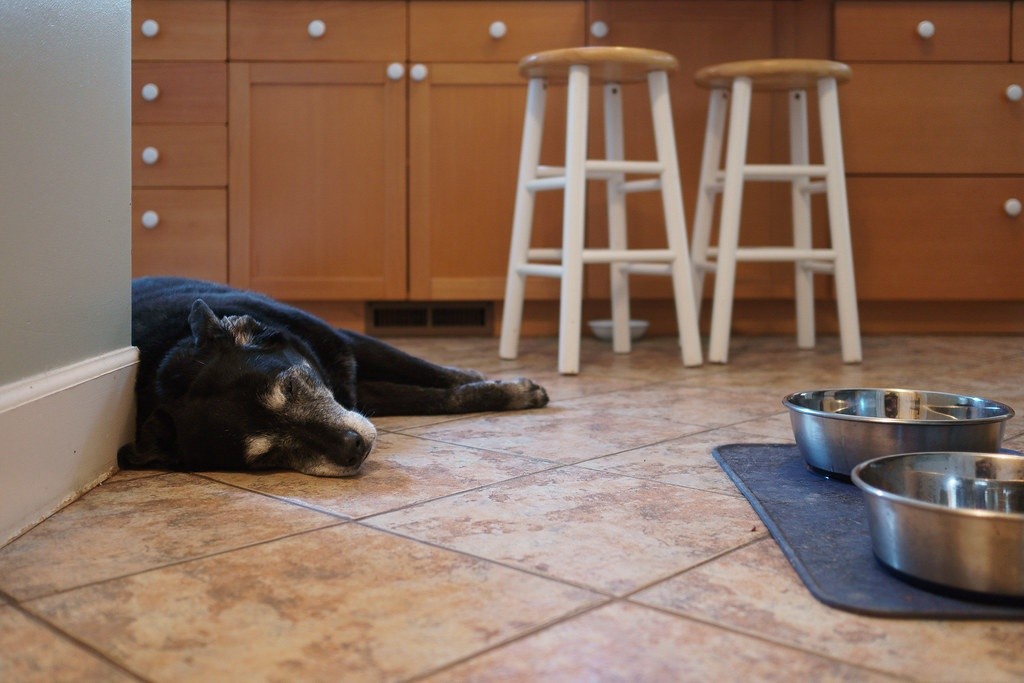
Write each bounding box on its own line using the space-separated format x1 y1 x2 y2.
782 387 1015 482
853 452 1023 602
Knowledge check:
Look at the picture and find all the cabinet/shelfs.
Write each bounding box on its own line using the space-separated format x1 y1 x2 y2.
584 1 840 336
833 2 1024 335
130 1 583 340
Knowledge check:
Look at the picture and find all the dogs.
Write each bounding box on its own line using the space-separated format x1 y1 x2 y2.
117 275 550 479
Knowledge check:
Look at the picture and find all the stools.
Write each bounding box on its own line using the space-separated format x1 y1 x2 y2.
689 59 864 365
497 46 705 374
